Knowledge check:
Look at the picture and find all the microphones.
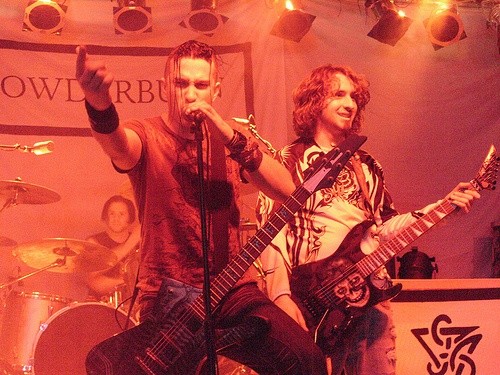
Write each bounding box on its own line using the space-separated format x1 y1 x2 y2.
33 141 56 156
189 110 204 120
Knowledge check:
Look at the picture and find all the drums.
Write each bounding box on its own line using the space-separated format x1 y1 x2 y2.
0 288 75 375
121 249 142 300
31 299 138 374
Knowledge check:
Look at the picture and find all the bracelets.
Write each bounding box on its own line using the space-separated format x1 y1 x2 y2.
225 129 262 184
84 98 119 135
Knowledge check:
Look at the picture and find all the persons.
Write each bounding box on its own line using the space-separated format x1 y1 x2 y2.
82 195 141 300
255 65 481 375
75 39 328 375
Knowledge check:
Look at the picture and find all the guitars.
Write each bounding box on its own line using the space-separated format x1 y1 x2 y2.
289 145 500 357
86 132 368 375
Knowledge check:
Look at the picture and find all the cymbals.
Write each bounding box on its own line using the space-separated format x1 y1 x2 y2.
0 179 61 205
14 237 119 274
0 235 17 247
